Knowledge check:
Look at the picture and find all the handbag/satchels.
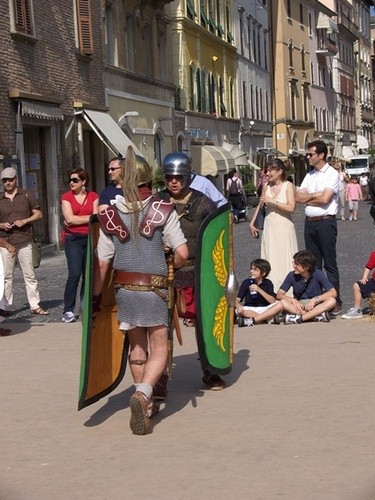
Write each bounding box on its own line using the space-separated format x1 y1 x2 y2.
31 233 42 269
60 230 65 244
254 206 265 230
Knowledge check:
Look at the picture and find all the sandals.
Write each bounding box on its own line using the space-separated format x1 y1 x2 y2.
0 309 11 316
31 307 49 315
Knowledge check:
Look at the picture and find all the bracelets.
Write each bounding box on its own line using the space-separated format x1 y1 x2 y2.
273 200 279 208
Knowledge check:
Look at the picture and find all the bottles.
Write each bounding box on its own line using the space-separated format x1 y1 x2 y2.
249 279 258 298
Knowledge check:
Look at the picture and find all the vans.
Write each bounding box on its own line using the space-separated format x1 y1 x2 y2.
344 154 373 183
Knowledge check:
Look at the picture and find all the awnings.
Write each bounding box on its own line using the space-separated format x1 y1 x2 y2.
357 135 369 148
342 145 360 158
81 108 143 160
189 142 249 176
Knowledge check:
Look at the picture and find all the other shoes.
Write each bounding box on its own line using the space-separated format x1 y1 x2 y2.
233 214 239 224
129 390 151 434
202 373 225 390
146 401 159 417
329 305 342 315
341 216 345 221
152 373 169 400
349 216 357 221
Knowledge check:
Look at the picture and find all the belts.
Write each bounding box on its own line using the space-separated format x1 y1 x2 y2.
112 269 169 289
306 214 335 222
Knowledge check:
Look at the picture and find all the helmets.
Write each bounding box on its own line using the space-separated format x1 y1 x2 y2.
120 145 152 249
163 151 193 177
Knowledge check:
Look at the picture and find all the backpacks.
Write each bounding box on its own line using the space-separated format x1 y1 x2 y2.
229 177 239 195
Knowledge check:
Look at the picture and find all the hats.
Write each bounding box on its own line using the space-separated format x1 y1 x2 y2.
0 167 18 180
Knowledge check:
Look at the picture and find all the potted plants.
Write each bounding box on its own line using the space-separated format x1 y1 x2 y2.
243 183 259 206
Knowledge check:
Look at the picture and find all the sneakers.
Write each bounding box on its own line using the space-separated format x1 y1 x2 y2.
314 310 331 322
342 307 364 319
266 314 280 325
62 311 76 323
237 316 256 327
284 313 302 325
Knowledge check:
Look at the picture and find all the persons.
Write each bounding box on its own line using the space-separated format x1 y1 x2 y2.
359 173 369 201
0 168 49 315
334 165 349 220
92 145 188 435
97 158 125 213
346 175 363 221
226 169 243 224
155 151 229 400
277 251 337 324
249 158 300 316
235 258 285 327
294 140 342 316
341 251 375 318
60 167 100 322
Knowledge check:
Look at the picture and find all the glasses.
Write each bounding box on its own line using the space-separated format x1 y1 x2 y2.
107 167 120 171
306 153 316 157
68 177 82 184
